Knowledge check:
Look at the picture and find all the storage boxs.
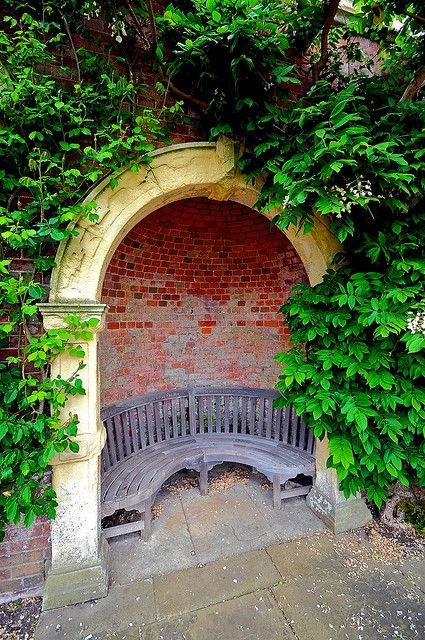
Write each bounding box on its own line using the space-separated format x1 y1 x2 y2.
98 383 320 541
